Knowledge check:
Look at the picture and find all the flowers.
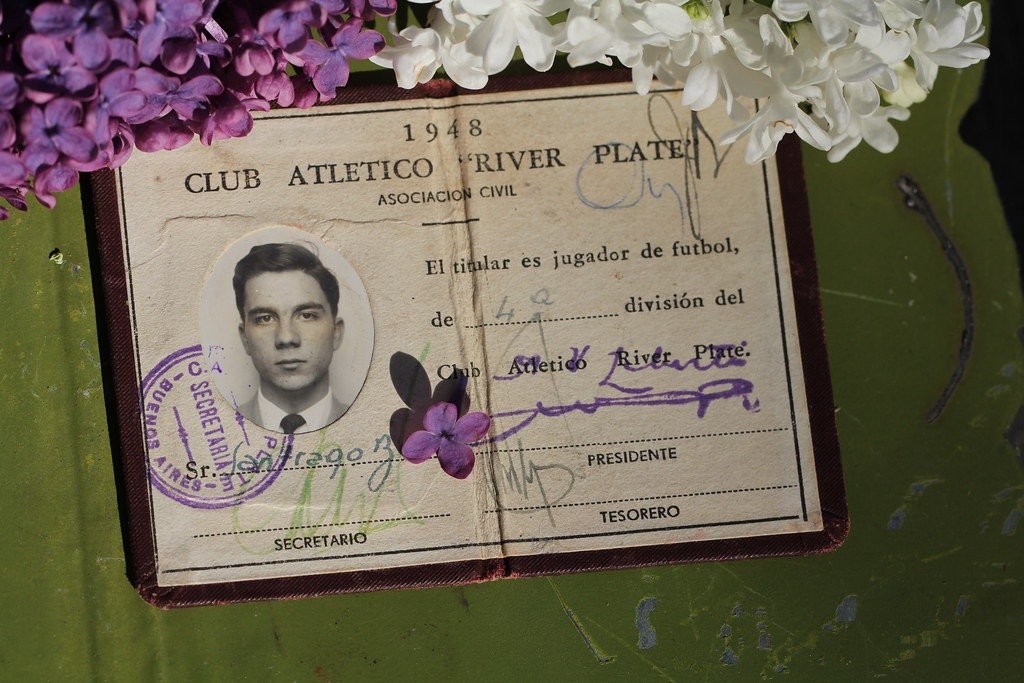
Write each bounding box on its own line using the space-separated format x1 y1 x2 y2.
0 0 991 222
400 401 490 480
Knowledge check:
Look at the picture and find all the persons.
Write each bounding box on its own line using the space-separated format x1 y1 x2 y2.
230 243 352 433
